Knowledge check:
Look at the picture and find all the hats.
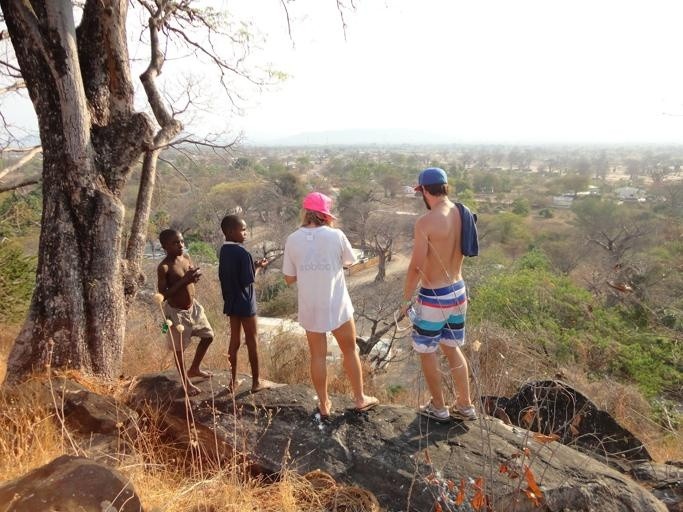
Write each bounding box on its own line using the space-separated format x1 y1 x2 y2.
303 192 339 220
414 167 449 192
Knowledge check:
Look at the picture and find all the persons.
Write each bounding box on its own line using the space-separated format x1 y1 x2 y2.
218 214 272 393
282 190 381 414
401 167 478 422
157 228 215 396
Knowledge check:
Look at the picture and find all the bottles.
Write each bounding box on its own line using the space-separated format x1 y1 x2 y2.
407 305 415 323
162 318 168 335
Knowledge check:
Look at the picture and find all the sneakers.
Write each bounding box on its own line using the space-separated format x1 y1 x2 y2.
415 396 450 421
449 399 478 420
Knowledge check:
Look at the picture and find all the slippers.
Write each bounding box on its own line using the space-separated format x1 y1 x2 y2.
357 396 380 413
318 399 332 418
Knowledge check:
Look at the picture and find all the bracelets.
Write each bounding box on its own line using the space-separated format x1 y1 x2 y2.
400 299 414 306
